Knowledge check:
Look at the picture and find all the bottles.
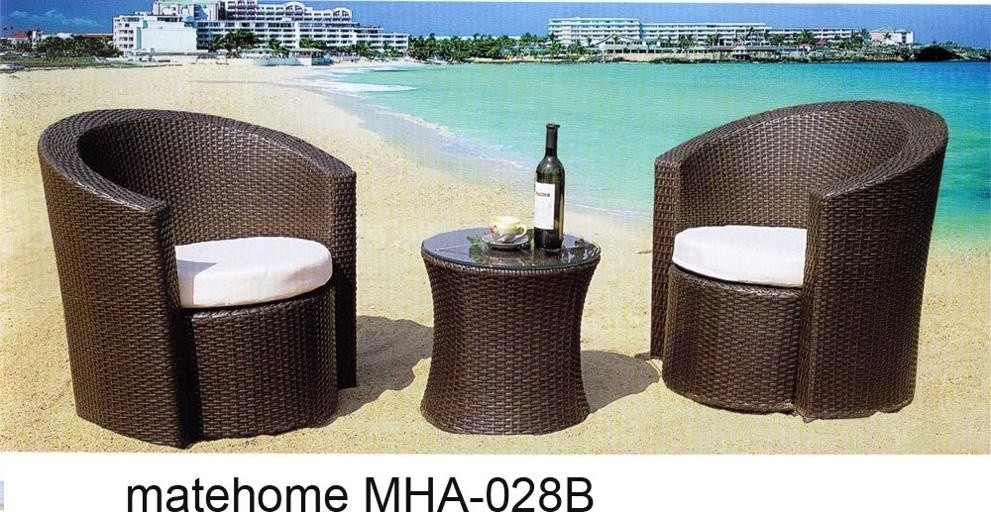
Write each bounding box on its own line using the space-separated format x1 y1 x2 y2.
534 124 564 253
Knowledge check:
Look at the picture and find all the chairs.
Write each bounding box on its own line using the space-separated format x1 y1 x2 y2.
37 107 357 449
649 98 949 420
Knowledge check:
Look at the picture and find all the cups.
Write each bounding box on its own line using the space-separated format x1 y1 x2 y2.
491 217 525 242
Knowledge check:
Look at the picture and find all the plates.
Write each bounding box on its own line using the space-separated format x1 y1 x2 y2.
482 234 528 249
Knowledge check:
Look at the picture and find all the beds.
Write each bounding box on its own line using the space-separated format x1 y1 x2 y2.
419 227 601 436
